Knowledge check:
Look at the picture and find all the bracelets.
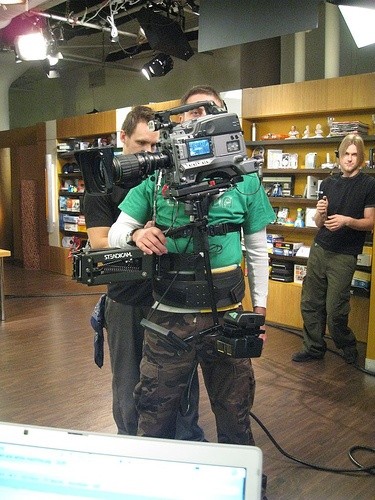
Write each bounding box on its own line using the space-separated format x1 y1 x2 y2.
126 227 143 248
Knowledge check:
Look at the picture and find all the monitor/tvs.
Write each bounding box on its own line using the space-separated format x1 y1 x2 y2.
0 422 262 500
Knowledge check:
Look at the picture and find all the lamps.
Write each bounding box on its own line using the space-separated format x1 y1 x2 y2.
140 54 175 81
137 1 194 62
42 59 62 79
11 15 60 64
46 30 64 65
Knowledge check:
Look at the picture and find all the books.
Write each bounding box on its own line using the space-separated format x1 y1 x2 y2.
57 142 74 153
328 120 370 138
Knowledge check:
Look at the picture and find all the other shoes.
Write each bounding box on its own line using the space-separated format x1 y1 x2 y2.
293 351 324 362
342 344 359 364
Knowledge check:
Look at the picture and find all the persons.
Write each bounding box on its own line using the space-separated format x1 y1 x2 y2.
291 133 375 364
78 105 211 445
107 84 270 500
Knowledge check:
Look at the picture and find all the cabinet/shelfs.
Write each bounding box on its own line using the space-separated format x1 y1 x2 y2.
235 71 375 343
57 109 128 280
